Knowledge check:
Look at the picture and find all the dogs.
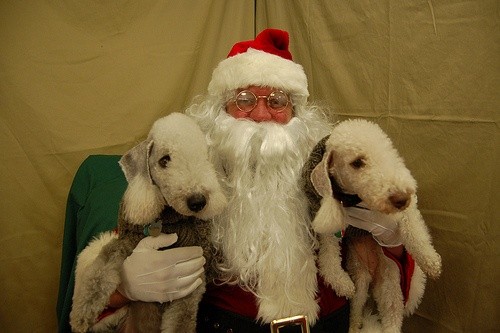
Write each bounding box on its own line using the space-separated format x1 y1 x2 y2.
302 118 443 333
68 111 230 333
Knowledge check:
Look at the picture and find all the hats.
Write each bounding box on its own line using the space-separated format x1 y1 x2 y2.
207 28 310 99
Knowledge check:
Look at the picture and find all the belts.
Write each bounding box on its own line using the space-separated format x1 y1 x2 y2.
195 297 351 333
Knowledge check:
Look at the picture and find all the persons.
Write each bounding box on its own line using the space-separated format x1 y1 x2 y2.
67 28 426 332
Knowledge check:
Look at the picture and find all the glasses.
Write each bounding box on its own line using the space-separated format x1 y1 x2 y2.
226 89 293 113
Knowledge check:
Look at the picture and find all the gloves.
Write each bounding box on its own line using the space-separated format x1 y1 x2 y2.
344 201 405 249
114 233 206 304
